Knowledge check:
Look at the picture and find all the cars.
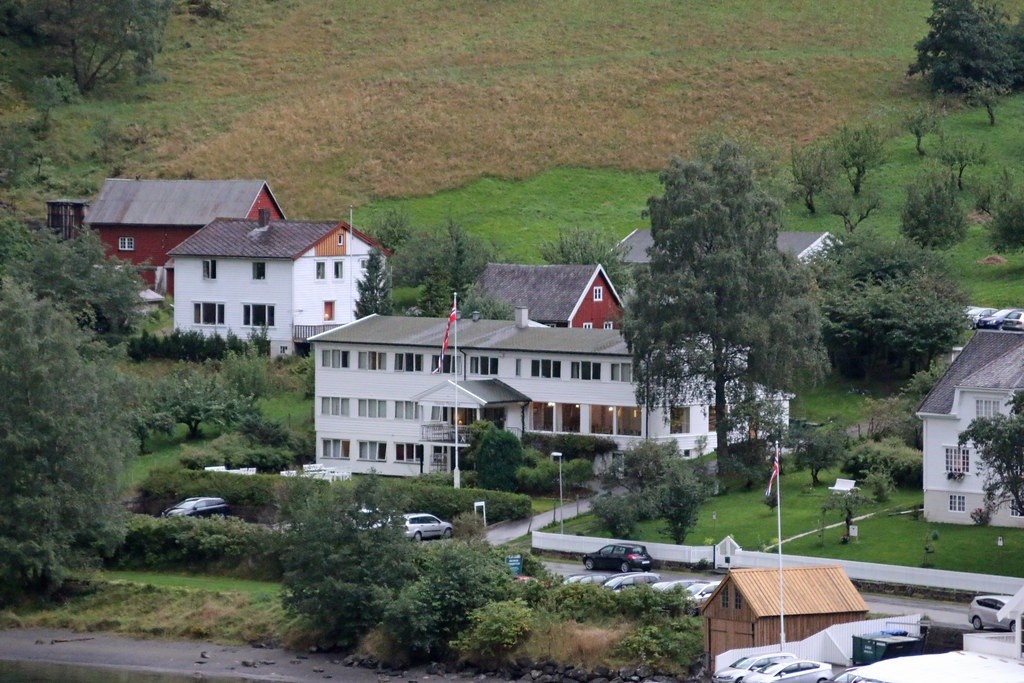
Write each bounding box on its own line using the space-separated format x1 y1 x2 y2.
582 543 653 572
964 305 1024 331
741 660 833 683
561 573 722 617
160 496 228 520
401 514 454 542
968 595 1024 632
711 652 799 683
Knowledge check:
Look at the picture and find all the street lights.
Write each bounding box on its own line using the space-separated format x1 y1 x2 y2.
550 452 564 535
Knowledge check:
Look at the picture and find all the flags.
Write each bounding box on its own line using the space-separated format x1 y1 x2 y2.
766 445 780 497
437 302 461 375
